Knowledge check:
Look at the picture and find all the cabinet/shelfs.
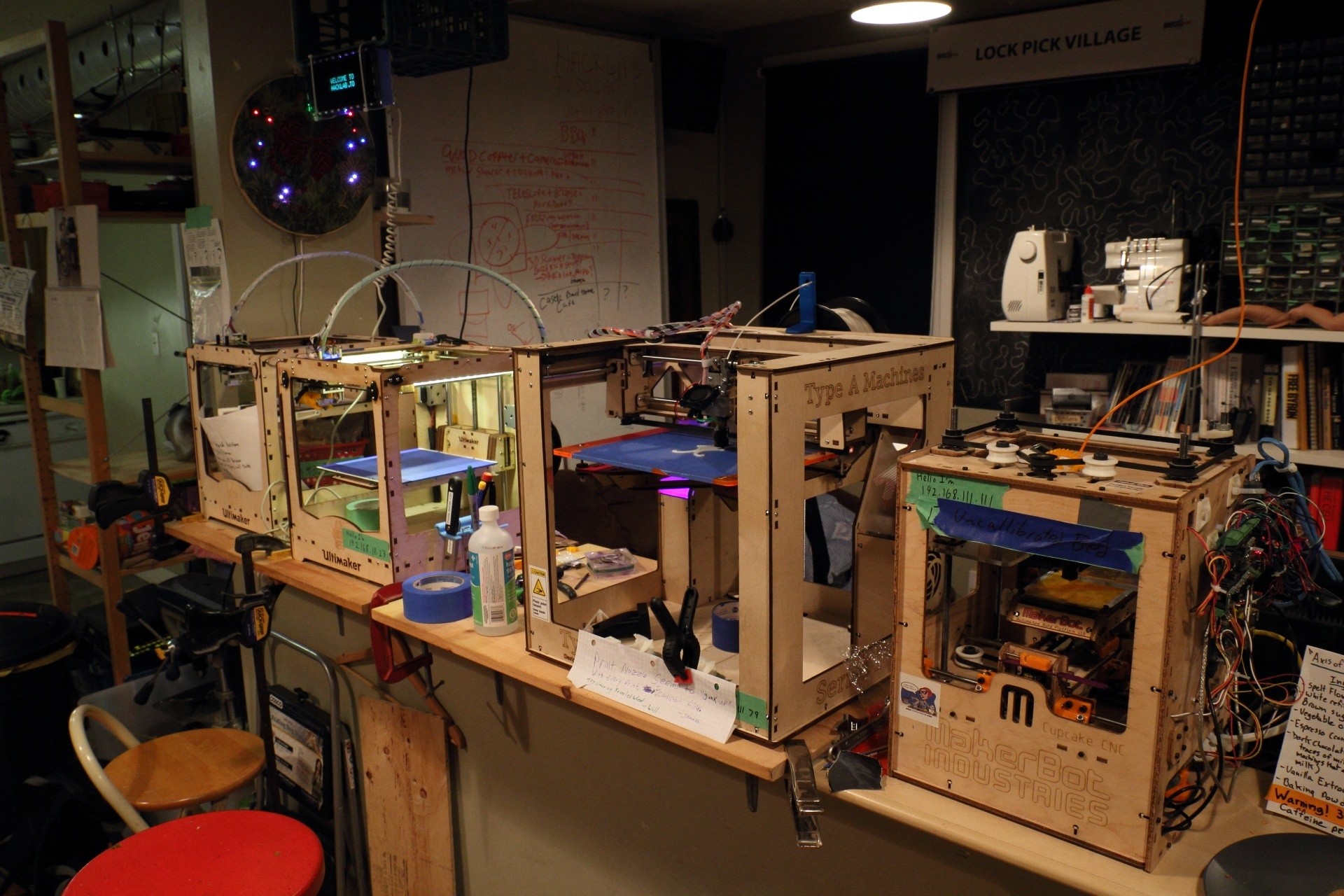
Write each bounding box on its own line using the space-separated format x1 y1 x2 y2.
988 321 1344 568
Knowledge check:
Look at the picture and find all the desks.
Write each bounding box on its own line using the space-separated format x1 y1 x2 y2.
161 502 1344 896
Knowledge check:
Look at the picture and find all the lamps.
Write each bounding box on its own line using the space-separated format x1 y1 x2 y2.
850 0 955 27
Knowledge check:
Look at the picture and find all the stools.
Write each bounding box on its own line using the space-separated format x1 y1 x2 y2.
68 704 267 832
60 810 325 896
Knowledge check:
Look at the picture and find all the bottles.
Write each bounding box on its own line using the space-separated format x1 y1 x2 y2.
467 505 519 637
1081 287 1095 324
